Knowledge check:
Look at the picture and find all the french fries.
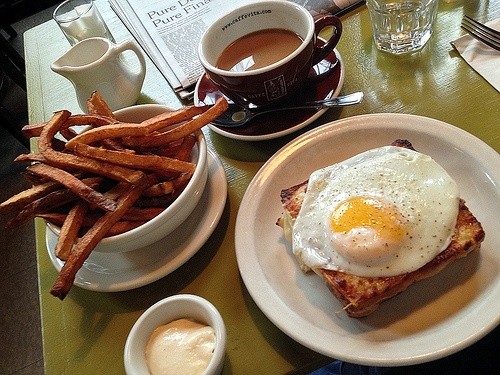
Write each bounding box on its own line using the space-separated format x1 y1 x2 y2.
0 91 229 300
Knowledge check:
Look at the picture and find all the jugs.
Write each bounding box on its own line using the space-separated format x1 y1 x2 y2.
50 37 146 114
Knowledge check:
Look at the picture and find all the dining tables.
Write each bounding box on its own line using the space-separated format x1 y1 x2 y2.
23 0 500 375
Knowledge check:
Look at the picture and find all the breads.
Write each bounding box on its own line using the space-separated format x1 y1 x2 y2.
277 139 486 318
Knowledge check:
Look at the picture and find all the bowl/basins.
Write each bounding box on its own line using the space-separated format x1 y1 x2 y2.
45 103 209 253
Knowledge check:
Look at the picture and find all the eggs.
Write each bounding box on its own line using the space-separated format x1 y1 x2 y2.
292 145 460 277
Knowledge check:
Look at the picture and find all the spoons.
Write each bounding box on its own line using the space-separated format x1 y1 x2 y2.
199 91 365 127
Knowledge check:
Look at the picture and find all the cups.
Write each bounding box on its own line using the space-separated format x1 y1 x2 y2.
52 0 117 47
366 0 439 55
198 1 343 113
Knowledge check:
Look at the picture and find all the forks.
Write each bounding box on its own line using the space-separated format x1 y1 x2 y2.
460 14 500 51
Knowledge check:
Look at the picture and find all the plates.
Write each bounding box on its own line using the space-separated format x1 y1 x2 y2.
123 294 227 375
193 35 344 141
46 147 227 293
234 112 499 367
